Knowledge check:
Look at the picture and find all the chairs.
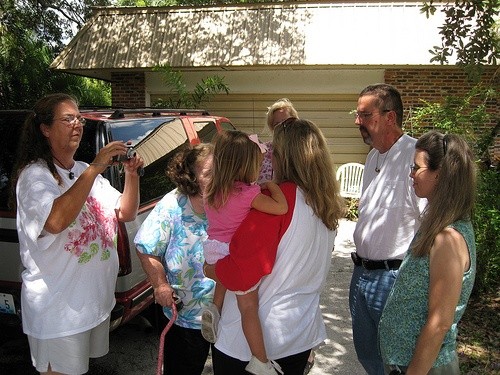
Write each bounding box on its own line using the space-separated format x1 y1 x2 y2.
335 162 365 198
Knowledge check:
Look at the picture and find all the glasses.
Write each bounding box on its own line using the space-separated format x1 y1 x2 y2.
51 117 86 126
409 164 443 173
281 118 297 135
354 110 392 119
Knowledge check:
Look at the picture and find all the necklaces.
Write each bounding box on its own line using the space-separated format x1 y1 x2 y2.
375 133 404 172
50 153 74 180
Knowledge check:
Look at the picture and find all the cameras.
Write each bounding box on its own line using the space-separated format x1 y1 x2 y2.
122 146 136 162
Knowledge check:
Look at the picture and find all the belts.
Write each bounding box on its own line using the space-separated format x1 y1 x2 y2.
356 252 403 272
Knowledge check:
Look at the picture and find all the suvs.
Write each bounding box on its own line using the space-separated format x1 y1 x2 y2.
0 107 239 329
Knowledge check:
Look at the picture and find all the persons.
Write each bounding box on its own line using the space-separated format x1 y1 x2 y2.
203 130 288 375
378 131 478 375
348 83 429 375
133 142 216 375
253 98 301 185
202 116 346 375
11 94 144 375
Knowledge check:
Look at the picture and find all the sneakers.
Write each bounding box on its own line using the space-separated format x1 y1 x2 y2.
246 356 283 375
201 308 219 343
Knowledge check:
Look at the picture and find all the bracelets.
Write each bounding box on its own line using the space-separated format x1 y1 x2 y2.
204 264 209 278
